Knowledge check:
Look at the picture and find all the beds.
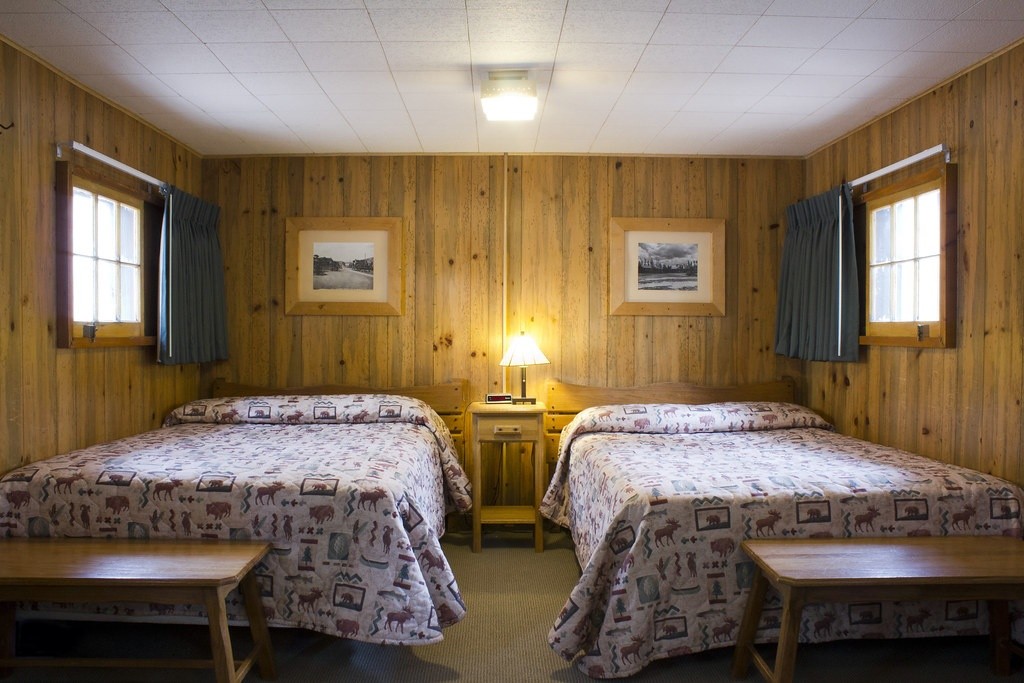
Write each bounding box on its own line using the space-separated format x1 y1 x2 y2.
0 378 474 648
538 374 1024 680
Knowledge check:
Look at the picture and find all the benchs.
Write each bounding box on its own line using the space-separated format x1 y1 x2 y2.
0 531 275 683
724 535 1024 683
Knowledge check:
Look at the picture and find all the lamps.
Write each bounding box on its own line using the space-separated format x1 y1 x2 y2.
470 69 550 128
496 328 554 406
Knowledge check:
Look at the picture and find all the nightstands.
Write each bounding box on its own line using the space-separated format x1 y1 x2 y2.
467 403 550 557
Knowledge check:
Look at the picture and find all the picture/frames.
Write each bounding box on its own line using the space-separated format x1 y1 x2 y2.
607 216 728 321
284 212 405 318
